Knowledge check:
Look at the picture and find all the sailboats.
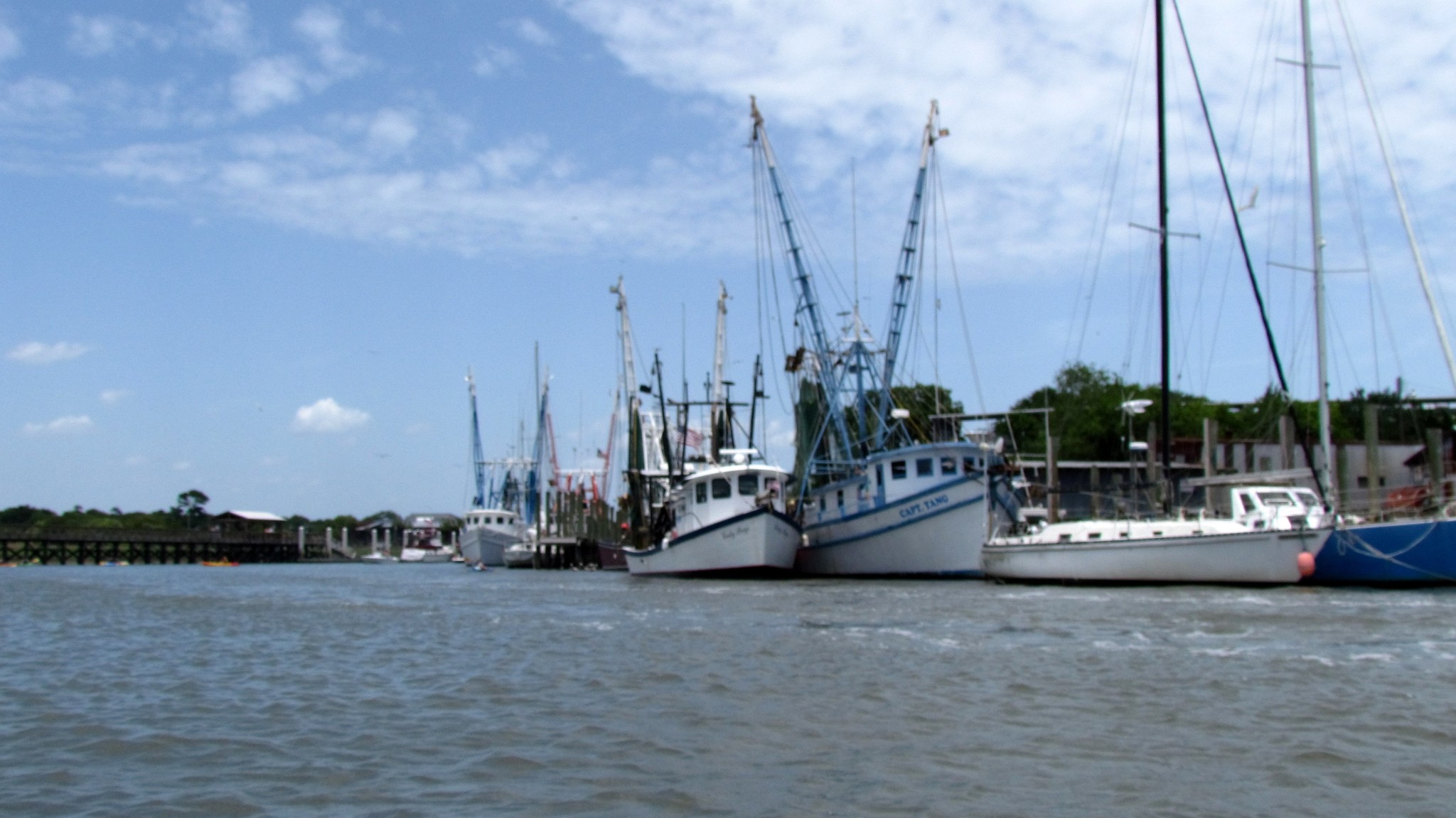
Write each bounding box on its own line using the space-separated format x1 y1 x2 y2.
742 92 1024 576
1294 1 1455 587
452 1 809 579
984 0 1338 585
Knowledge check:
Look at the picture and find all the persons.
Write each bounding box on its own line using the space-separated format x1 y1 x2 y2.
755 486 779 507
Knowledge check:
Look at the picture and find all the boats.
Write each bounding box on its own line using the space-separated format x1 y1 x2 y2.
400 527 453 563
361 551 398 563
99 561 129 567
202 560 238 567
0 558 41 569
